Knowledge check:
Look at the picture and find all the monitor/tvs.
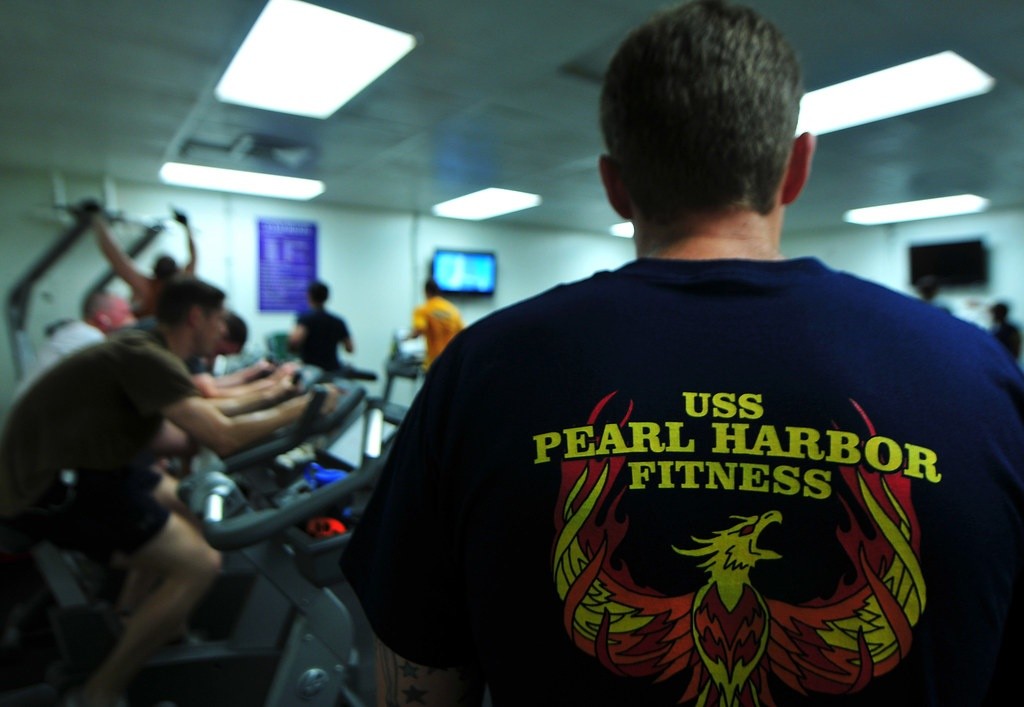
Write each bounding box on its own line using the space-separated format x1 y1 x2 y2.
432 248 497 296
909 239 987 289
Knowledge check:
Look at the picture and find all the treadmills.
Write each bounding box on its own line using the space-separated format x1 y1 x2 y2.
266 328 430 412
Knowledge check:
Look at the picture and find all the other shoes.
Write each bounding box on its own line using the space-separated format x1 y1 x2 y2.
61 688 128 707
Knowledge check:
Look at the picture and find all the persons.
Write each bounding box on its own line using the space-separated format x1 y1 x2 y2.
0 197 355 707
337 0 1024 707
